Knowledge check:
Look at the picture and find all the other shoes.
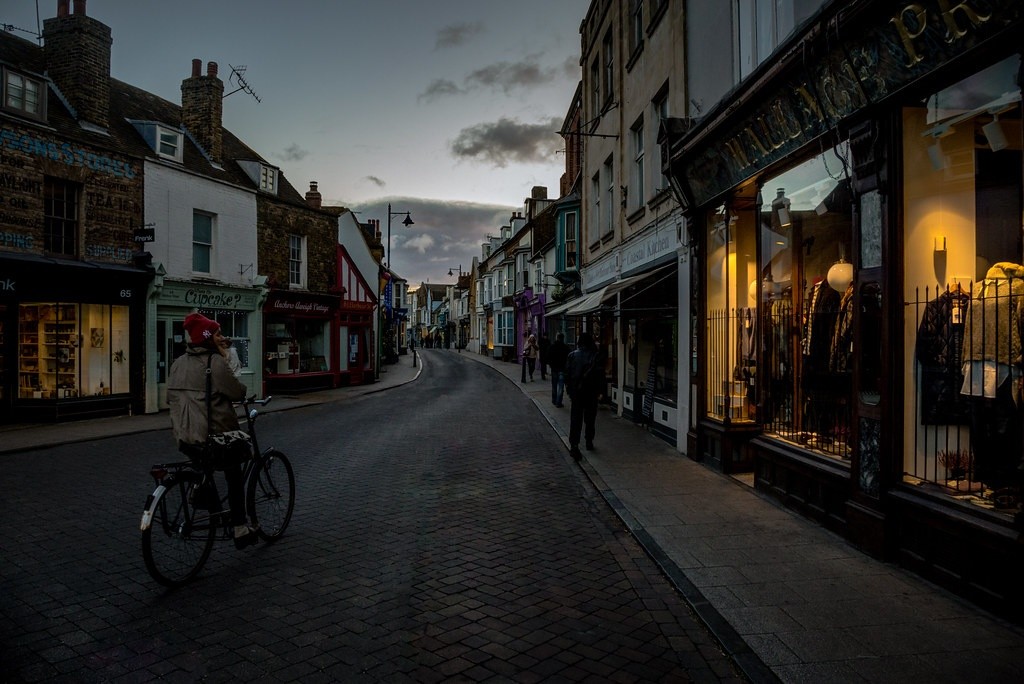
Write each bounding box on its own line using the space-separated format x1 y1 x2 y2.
234 525 262 549
555 404 564 407
192 495 208 509
529 375 533 382
553 402 556 405
569 448 583 461
587 444 597 450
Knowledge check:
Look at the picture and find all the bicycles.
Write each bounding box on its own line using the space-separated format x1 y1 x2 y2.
139 394 296 588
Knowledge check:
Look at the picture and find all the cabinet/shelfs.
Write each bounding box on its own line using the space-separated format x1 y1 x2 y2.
18 303 80 398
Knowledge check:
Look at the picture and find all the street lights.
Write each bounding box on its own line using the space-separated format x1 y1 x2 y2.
387 203 416 361
448 264 461 353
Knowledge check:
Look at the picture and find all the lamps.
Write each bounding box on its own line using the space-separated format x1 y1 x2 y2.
827 258 854 293
777 202 791 227
921 98 1010 170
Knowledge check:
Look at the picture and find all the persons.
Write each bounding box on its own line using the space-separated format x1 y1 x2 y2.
165 313 261 539
220 338 241 377
547 333 572 408
420 332 442 348
524 334 539 381
537 333 551 380
565 333 608 460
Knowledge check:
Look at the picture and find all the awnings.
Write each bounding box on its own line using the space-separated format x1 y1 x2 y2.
544 290 598 317
429 326 437 333
565 262 678 316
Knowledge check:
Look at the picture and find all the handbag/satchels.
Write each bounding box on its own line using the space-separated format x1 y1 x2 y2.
523 346 531 356
205 429 254 471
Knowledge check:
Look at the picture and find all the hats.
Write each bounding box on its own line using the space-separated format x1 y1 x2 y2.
183 313 219 343
